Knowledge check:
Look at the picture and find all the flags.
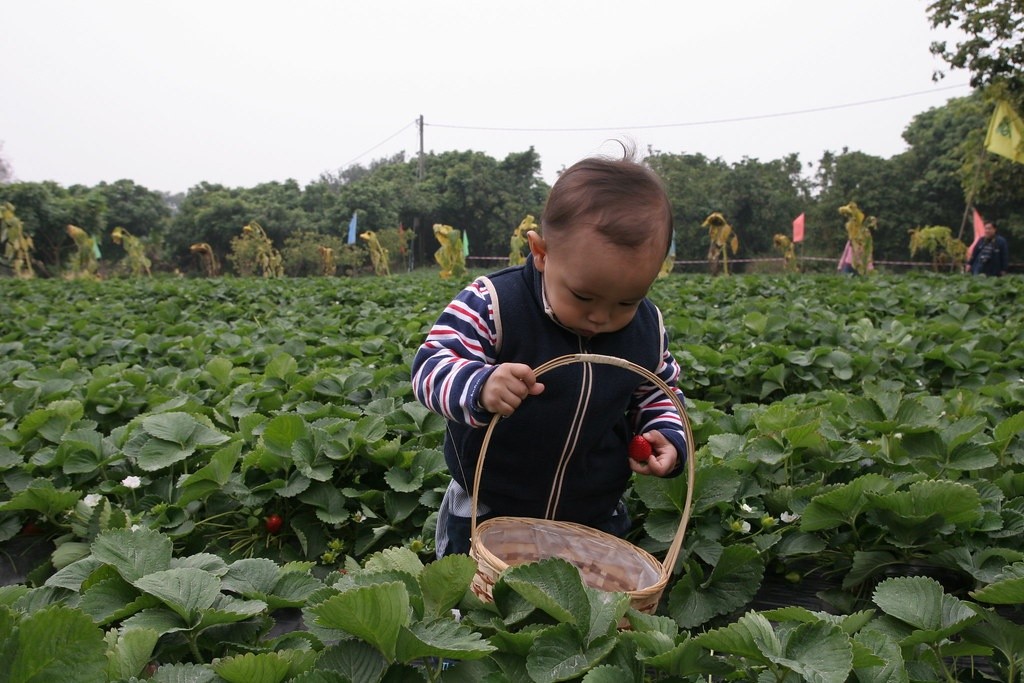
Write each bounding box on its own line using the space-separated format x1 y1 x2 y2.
983 101 1024 164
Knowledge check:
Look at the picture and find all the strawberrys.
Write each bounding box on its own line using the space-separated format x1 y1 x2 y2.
628 434 652 462
266 514 282 532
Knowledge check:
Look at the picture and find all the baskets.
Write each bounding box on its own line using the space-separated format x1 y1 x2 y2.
468 353 694 634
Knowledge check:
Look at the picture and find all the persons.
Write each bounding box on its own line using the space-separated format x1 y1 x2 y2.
412 135 694 612
837 231 873 274
967 218 1007 277
